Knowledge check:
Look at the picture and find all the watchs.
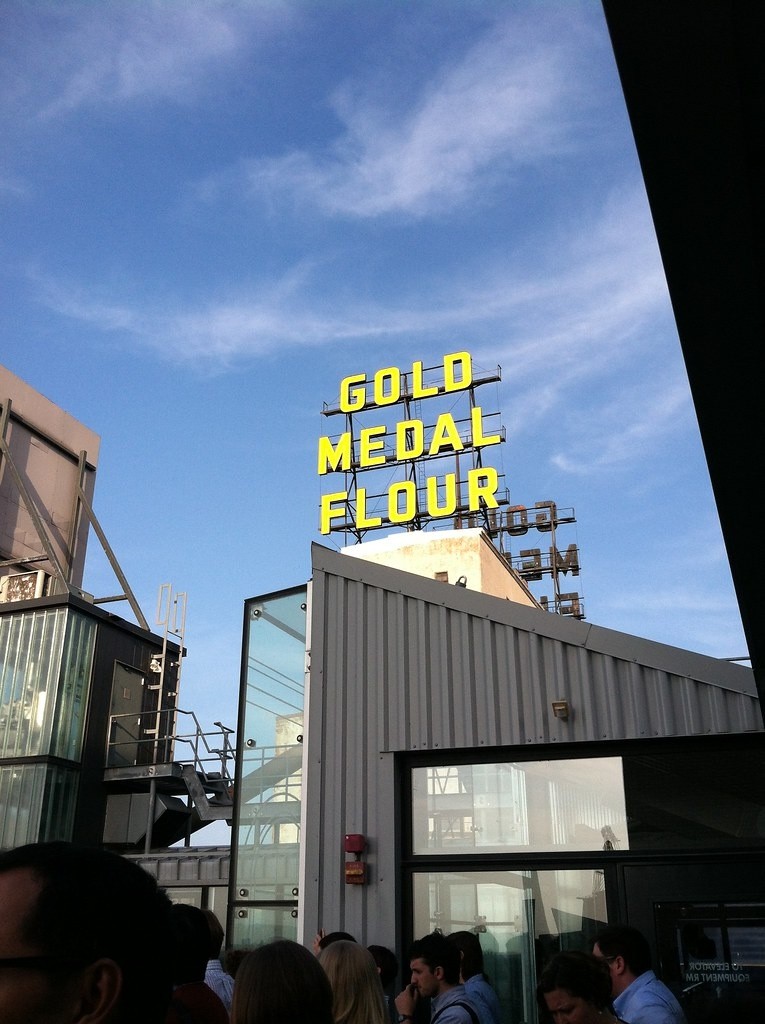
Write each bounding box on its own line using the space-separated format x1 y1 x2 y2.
397 1015 413 1023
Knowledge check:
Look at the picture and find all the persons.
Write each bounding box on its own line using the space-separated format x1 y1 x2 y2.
0 841 688 1024
